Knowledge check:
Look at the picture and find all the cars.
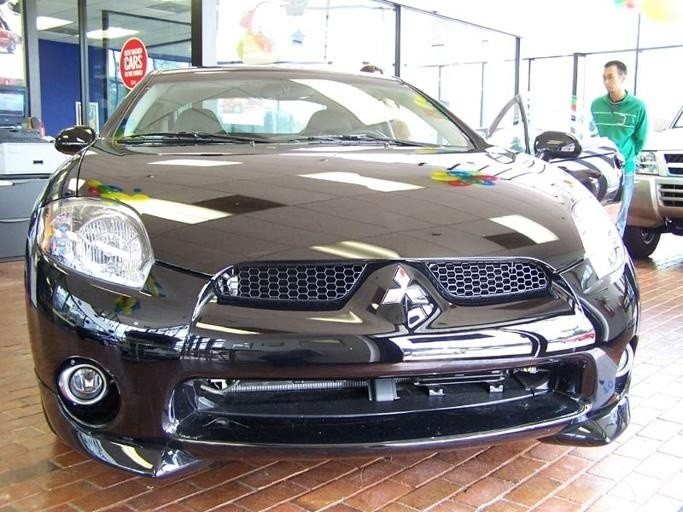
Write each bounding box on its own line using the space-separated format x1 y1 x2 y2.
0 15 22 52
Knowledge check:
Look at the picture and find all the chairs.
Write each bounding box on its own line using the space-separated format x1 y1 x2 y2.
303 109 352 136
169 108 224 134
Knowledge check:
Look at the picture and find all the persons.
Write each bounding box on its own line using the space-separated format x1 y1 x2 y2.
360 65 383 73
591 60 648 240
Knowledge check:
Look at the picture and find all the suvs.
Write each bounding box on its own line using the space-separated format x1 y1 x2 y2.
25 70 639 478
623 107 683 258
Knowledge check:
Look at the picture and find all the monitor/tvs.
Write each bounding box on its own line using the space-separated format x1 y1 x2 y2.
0 85 28 116
